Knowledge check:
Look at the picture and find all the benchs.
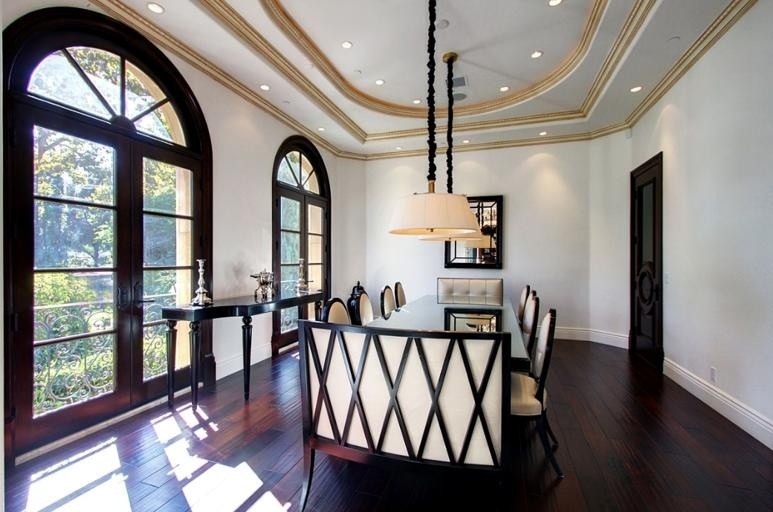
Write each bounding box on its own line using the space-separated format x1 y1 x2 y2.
437 277 503 296
298 318 513 512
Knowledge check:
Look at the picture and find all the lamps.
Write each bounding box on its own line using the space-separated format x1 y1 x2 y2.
421 53 480 241
463 203 496 249
387 0 480 239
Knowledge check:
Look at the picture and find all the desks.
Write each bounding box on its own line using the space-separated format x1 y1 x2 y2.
161 291 323 411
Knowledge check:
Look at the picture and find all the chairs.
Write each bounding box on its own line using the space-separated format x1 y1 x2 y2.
323 282 408 323
511 285 567 479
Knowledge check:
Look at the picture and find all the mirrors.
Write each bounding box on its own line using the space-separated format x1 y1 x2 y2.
444 194 502 269
444 308 502 332
